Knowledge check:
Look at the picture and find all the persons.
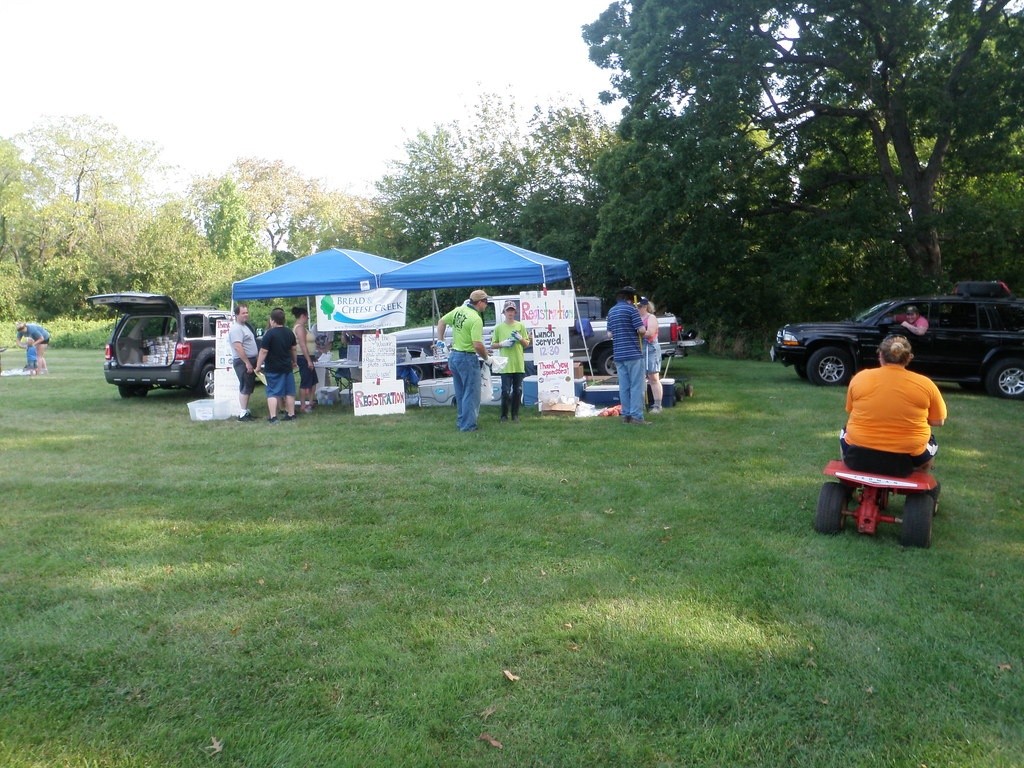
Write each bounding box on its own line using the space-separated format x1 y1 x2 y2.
887 306 929 335
437 290 509 431
492 300 529 420
311 321 334 385
255 307 297 423
290 307 319 413
340 330 364 360
838 334 949 477
605 286 663 426
227 304 259 422
20 338 40 375
14 322 50 375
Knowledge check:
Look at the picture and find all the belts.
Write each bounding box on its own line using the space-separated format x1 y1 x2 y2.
451 348 476 355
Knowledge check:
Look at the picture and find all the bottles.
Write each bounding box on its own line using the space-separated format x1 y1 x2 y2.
419 347 426 361
405 349 413 363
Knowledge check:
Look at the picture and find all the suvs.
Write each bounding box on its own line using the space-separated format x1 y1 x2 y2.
87 294 267 398
771 281 1024 398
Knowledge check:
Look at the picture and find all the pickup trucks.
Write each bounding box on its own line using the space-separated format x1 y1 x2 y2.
387 295 688 385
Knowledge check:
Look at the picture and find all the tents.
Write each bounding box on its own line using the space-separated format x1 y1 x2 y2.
230 245 435 379
379 237 597 385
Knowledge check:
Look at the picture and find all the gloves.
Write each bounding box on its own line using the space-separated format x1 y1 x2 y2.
511 331 522 340
499 338 516 348
485 355 492 366
437 340 445 348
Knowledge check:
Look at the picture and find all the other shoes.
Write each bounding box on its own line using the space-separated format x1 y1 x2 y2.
501 416 507 421
650 406 663 414
631 418 652 425
279 414 296 421
279 410 287 415
623 415 632 423
267 416 279 423
300 404 315 412
513 415 520 423
238 413 256 422
293 410 297 414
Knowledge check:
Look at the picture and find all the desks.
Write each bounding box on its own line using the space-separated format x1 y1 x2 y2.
396 352 574 379
313 361 362 369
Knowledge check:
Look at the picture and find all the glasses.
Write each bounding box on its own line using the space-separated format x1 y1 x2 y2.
883 334 908 341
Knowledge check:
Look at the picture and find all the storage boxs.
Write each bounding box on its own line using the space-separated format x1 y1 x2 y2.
575 375 587 399
585 384 625 404
317 385 339 405
339 389 353 407
416 378 456 408
142 332 179 365
187 398 232 421
648 378 676 407
521 375 538 408
480 376 503 405
573 361 584 378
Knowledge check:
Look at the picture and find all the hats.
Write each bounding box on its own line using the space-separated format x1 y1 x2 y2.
504 301 516 311
470 290 493 302
637 296 648 307
615 285 642 302
906 306 917 314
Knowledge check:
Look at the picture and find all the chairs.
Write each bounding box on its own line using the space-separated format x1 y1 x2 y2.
333 346 352 390
396 346 417 393
